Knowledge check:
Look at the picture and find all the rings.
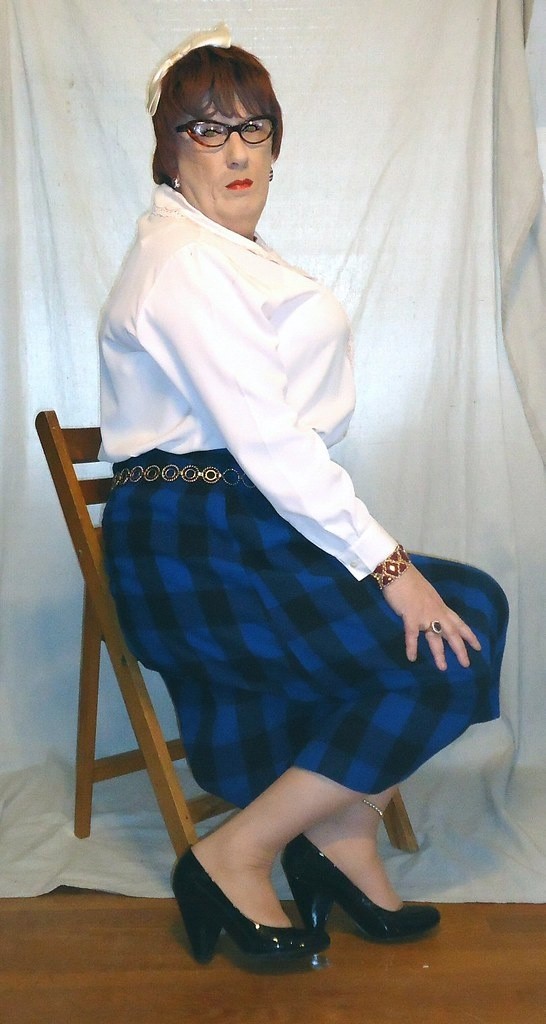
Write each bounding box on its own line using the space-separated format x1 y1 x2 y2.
423 619 443 634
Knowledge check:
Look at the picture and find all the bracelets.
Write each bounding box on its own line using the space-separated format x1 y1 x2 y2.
371 545 412 592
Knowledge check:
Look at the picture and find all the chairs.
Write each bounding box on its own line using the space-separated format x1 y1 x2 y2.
33 409 421 863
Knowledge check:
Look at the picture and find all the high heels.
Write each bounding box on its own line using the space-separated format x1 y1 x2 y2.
172 845 332 963
281 832 441 943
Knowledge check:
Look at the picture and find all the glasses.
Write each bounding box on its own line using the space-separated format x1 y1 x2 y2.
175 115 278 147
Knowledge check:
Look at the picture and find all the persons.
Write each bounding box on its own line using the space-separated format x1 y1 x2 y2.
98 20 509 963
